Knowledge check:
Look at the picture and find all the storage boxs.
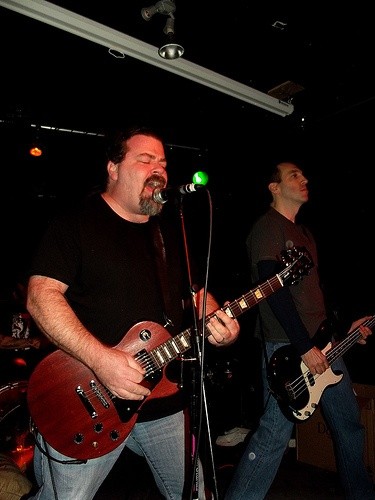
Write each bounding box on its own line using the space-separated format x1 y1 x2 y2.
296 383 375 482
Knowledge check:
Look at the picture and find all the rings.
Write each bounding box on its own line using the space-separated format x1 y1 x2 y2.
218 338 224 343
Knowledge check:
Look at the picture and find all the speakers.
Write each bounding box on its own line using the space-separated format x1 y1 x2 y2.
294 383 375 481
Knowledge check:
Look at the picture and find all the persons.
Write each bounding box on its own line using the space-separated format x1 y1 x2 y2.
0 278 49 362
221 163 375 500
27 126 240 500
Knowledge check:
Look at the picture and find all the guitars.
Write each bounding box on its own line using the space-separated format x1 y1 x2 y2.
268 316 375 423
26 246 314 459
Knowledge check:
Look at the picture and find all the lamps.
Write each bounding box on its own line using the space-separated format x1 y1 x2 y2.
30 124 42 155
140 0 184 59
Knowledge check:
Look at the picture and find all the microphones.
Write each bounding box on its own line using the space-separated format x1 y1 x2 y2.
151 183 204 205
